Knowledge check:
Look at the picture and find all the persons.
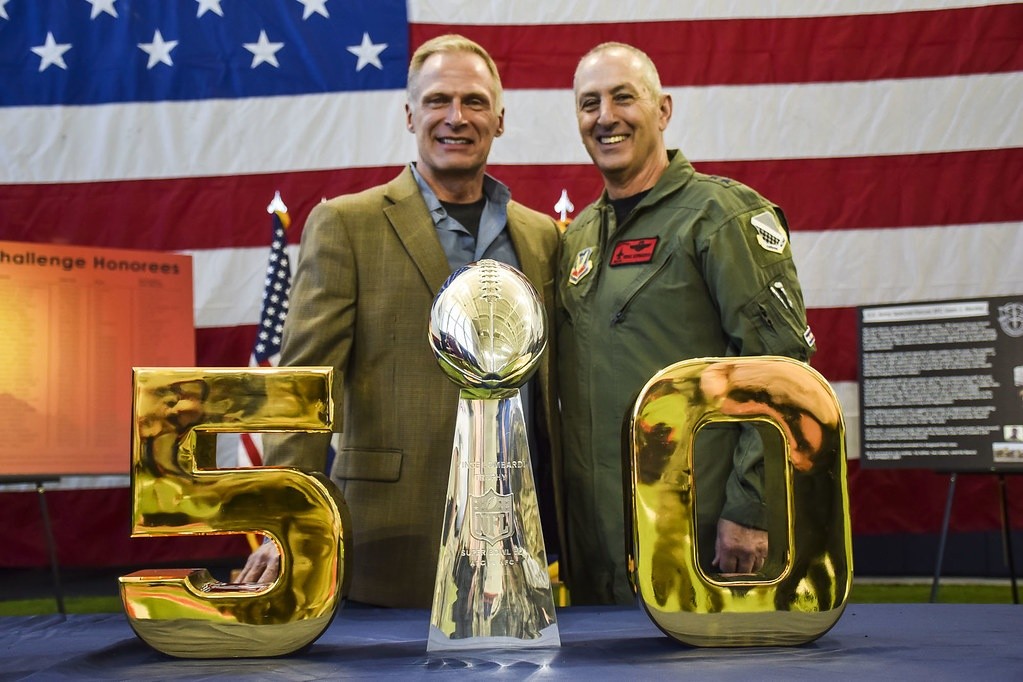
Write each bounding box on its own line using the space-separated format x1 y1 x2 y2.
550 40 818 606
232 31 565 610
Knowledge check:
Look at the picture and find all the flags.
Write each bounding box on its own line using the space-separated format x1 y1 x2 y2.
0 4 1023 567
235 207 292 469
